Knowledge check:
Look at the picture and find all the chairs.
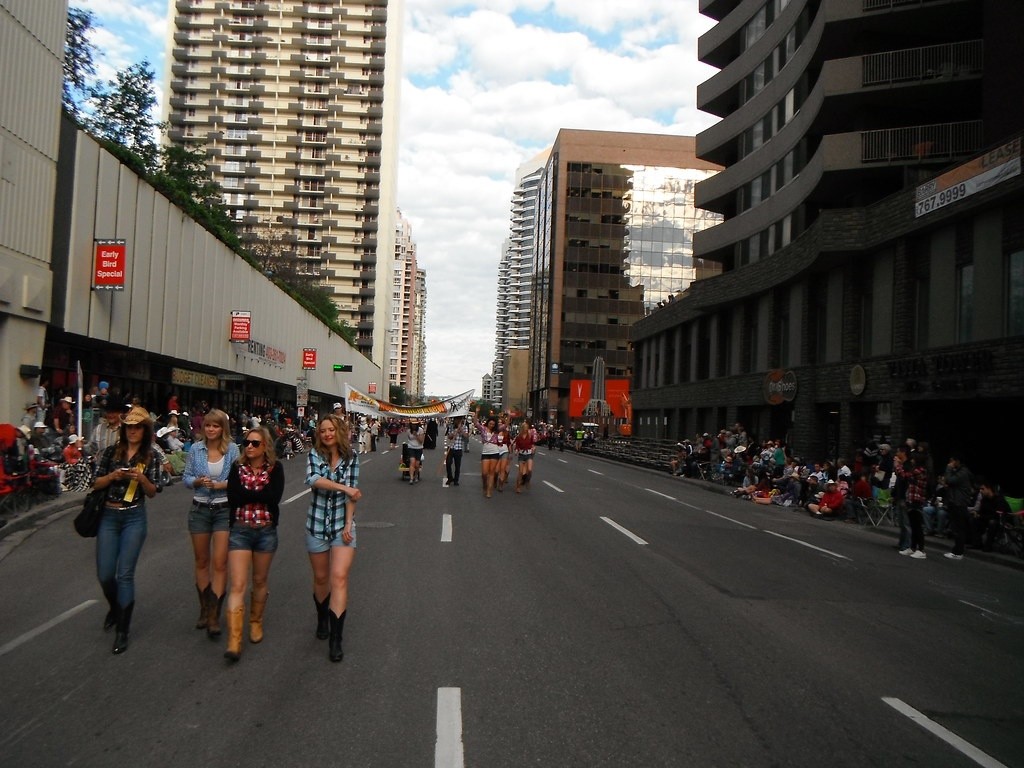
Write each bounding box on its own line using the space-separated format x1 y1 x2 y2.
0 424 59 517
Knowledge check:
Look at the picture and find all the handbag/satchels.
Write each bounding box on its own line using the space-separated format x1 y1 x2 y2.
74 488 108 538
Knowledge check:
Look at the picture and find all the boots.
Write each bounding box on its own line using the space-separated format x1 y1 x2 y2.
112 599 134 653
328 608 346 662
193 581 227 635
312 592 331 640
250 590 270 643
103 580 119 632
223 605 245 659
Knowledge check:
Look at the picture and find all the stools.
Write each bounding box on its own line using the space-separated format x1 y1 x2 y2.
697 449 719 480
858 489 894 528
1004 497 1023 544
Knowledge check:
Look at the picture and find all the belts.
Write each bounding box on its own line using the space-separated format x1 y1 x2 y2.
192 499 229 510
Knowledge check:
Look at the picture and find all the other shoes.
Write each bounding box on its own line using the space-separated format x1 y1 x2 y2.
943 552 963 560
409 477 418 485
445 476 459 485
898 547 926 558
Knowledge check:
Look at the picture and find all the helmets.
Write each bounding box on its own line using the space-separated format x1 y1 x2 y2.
360 417 367 424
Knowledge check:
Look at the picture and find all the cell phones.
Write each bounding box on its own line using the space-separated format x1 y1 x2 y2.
120 468 130 473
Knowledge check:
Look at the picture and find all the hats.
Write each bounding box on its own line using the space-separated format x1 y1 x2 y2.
22 400 40 410
723 456 733 462
155 427 180 437
59 397 76 405
734 446 746 453
808 473 818 479
168 409 180 416
68 434 85 444
703 432 709 436
823 478 838 490
683 439 690 443
765 440 774 445
789 473 801 481
410 418 419 424
333 403 342 410
33 421 47 428
101 393 129 411
118 406 153 427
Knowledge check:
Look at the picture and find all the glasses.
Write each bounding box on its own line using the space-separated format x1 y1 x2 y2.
242 440 263 447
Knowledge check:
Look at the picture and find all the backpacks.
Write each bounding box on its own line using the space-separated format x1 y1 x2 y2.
3 436 29 476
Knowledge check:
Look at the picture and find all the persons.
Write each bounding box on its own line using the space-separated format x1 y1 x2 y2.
223 428 286 661
304 415 359 663
182 409 240 638
21 393 1011 560
89 406 164 655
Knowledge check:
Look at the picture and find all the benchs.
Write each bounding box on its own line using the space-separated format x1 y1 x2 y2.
581 435 679 470
